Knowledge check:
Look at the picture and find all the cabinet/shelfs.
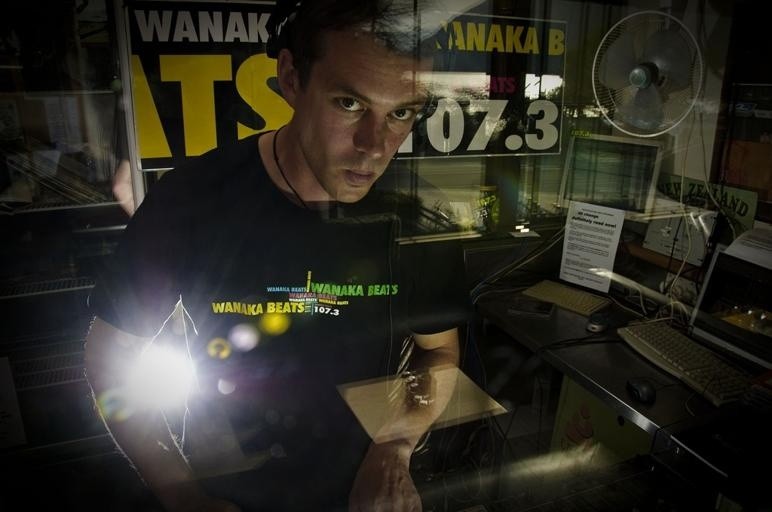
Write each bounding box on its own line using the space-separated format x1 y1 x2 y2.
0 1 154 452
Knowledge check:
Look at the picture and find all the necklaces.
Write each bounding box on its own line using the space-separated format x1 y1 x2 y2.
273 125 317 214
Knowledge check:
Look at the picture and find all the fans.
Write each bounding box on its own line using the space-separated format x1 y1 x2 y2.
591 10 705 137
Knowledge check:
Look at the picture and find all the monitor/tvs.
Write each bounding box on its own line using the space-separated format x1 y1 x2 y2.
689 242 772 371
557 132 664 224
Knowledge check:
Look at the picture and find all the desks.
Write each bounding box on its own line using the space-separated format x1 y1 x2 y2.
478 287 771 481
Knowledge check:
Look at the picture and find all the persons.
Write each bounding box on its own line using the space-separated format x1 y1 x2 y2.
83 2 473 510
109 96 136 218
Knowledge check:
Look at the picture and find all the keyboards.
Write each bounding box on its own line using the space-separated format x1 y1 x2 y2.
522 279 612 317
616 320 758 408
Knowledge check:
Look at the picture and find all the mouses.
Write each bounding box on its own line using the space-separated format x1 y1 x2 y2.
625 378 656 407
585 316 609 333
